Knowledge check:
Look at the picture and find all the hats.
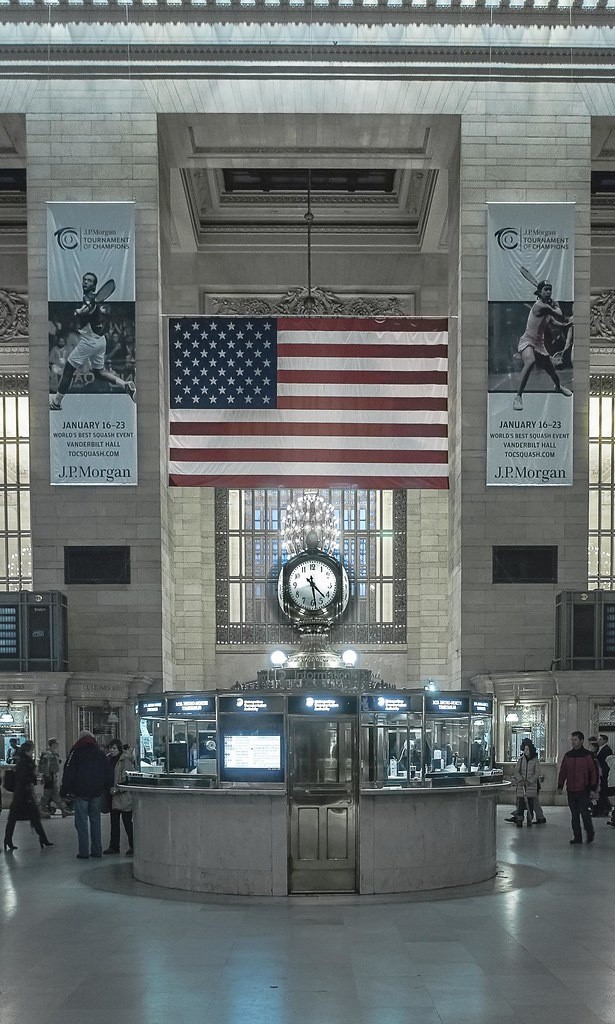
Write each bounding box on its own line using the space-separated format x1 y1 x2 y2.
534 280 552 295
49 738 58 745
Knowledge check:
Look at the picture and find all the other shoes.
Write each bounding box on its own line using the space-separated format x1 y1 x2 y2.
77 854 89 858
513 396 523 411
125 381 137 403
505 817 517 823
587 833 594 843
90 853 101 858
50 401 62 411
517 821 523 827
532 818 546 824
40 810 50 818
570 839 582 844
527 821 532 827
126 849 134 855
607 821 615 826
103 847 120 854
62 810 75 818
554 384 572 396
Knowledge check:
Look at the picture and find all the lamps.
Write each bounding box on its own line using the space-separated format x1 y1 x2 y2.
610 698 615 718
505 700 520 722
341 649 357 668
282 167 340 557
271 651 285 668
104 700 119 723
0 699 14 723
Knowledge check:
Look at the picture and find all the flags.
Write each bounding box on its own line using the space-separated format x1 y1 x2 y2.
168 316 450 488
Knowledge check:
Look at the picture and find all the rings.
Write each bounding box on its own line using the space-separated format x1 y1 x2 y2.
114 791 115 792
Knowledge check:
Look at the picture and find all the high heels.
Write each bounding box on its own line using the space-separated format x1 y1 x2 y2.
4 837 17 850
39 836 54 848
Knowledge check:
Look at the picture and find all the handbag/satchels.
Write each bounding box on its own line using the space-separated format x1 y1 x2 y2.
3 769 17 792
99 787 112 814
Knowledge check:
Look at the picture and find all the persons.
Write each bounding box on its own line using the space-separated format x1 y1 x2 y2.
446 745 455 764
556 731 600 844
104 745 109 754
587 735 612 817
154 736 166 757
6 739 19 764
412 739 431 769
49 272 136 410
60 729 108 859
473 745 482 762
606 754 615 826
124 744 132 754
40 738 71 817
504 738 546 828
4 742 54 849
513 280 574 411
103 739 133 855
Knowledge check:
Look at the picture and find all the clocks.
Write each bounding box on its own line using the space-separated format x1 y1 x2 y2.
277 551 349 616
